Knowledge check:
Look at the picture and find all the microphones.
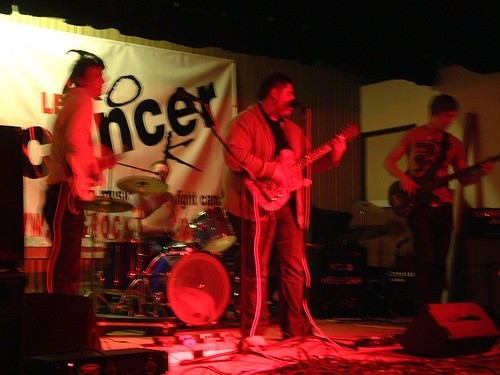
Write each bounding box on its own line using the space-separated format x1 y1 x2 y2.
290 99 309 110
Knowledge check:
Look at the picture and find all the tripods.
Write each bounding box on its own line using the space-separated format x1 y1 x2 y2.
116 185 168 316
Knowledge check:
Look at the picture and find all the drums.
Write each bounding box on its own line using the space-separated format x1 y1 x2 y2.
142 249 232 326
103 241 152 291
188 207 237 254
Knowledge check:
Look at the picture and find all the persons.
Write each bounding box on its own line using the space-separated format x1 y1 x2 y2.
43 59 120 297
130 161 179 232
384 93 493 313
220 72 347 348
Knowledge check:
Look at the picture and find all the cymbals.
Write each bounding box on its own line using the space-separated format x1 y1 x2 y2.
117 175 169 195
83 196 133 214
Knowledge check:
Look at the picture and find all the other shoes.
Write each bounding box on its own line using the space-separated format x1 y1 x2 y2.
242 335 266 348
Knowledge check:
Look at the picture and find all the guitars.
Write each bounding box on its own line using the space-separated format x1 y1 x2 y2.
244 124 361 213
388 155 500 218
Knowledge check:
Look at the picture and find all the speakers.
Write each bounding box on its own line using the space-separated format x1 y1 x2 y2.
20 293 104 356
400 302 499 360
